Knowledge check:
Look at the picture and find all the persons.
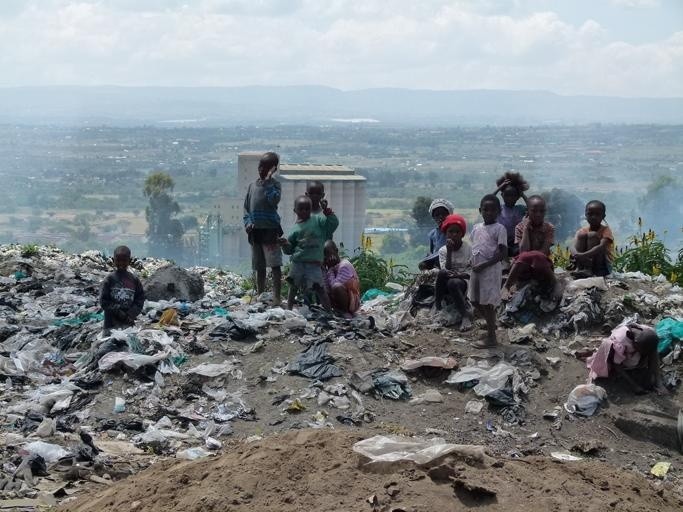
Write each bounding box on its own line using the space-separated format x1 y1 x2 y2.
490 172 529 273
275 195 339 315
98 245 145 337
316 239 361 319
565 199 615 277
408 198 455 320
468 194 508 350
581 320 665 397
302 179 339 308
431 214 475 333
500 194 564 308
241 151 284 308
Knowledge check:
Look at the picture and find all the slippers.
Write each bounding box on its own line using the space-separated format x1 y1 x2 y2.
469 339 498 348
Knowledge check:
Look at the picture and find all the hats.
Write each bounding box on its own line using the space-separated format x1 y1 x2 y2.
428 197 455 217
439 214 468 236
